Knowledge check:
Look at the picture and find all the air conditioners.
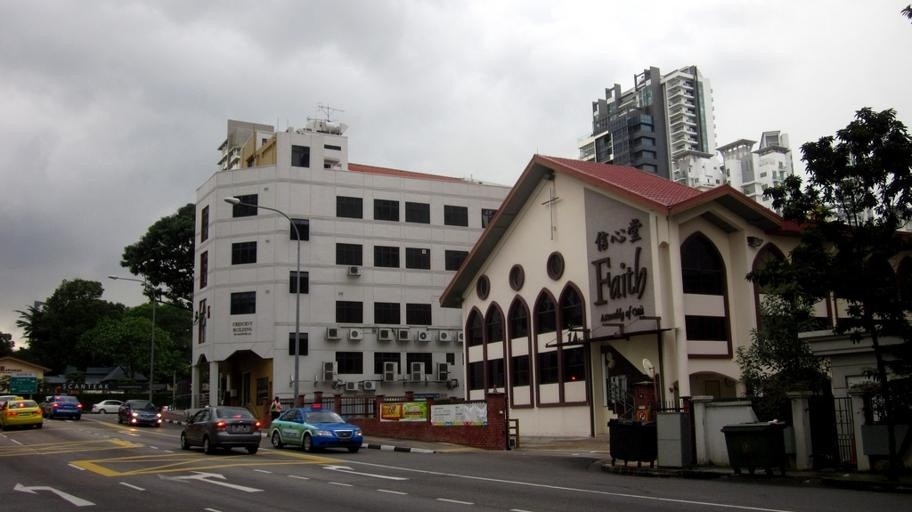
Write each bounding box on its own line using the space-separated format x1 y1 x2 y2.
363 380 376 391
347 264 362 278
417 331 433 342
345 382 359 391
456 331 463 343
378 328 394 341
398 328 410 341
382 361 398 383
438 329 452 341
437 362 450 382
348 327 363 340
410 361 426 382
326 327 342 340
321 361 338 383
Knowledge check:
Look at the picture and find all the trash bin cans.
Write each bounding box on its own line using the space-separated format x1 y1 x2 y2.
720 421 786 477
607 419 658 468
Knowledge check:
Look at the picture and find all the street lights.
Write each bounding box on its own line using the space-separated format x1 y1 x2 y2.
224 195 299 408
108 274 156 401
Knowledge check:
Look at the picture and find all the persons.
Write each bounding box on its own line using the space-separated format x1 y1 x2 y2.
269 396 283 422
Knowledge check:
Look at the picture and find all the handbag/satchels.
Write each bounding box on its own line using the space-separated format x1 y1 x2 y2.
271 401 276 409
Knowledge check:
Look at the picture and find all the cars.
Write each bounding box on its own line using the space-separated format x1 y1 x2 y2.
91 399 162 427
181 406 362 454
0 395 82 430
